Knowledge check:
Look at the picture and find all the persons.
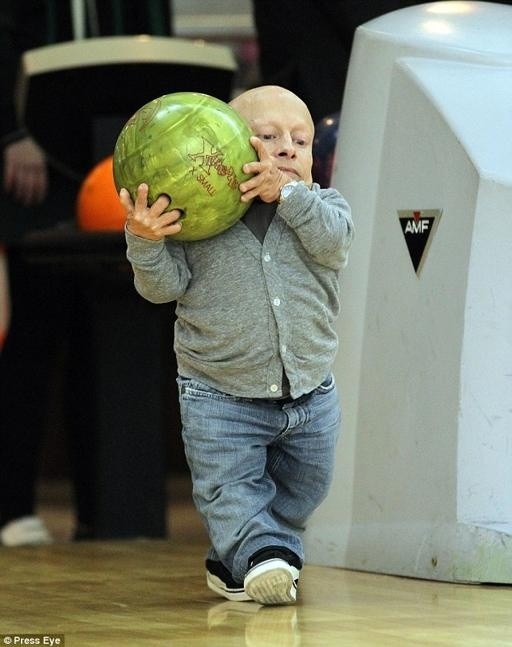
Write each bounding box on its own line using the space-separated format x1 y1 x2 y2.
3 1 58 553
114 83 352 606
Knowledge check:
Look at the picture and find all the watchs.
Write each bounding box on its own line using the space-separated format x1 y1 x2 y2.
278 180 298 204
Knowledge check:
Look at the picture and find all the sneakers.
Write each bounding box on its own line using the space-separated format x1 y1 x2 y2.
205 546 301 606
